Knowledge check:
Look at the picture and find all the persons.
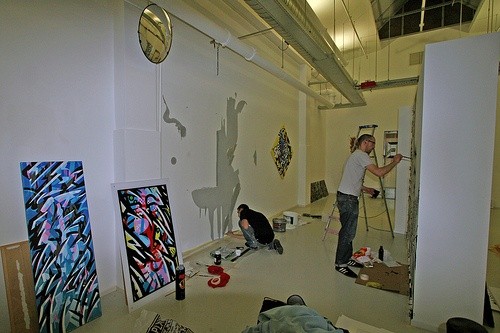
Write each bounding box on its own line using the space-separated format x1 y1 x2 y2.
237 203 283 255
334 134 402 278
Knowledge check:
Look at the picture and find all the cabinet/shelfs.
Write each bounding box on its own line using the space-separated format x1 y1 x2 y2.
383 34 500 333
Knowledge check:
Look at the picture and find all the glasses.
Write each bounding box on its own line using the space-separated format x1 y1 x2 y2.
367 139 375 145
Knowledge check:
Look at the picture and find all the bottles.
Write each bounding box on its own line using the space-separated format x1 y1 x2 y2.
379 246 384 261
214 251 221 265
176 265 185 300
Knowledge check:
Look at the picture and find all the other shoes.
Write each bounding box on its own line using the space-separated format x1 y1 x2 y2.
273 239 283 255
245 242 256 250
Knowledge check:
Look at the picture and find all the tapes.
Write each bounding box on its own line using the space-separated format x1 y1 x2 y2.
360 274 369 281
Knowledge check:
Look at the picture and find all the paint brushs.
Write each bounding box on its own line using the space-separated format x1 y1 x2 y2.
190 271 200 277
196 263 208 267
165 291 176 297
401 155 411 159
188 271 193 277
199 275 214 277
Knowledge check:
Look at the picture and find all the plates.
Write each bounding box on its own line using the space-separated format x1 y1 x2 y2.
357 256 370 263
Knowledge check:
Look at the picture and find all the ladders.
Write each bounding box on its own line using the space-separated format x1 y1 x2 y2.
325 124 394 238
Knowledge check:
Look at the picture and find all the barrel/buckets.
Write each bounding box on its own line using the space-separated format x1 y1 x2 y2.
272 218 287 232
283 211 298 229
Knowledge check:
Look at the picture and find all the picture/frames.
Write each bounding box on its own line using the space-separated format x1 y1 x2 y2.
111 178 184 315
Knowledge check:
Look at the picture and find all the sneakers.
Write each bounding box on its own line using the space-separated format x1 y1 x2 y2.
346 260 363 268
335 266 357 278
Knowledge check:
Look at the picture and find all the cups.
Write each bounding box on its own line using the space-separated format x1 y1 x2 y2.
235 250 241 256
371 189 380 199
365 247 371 256
